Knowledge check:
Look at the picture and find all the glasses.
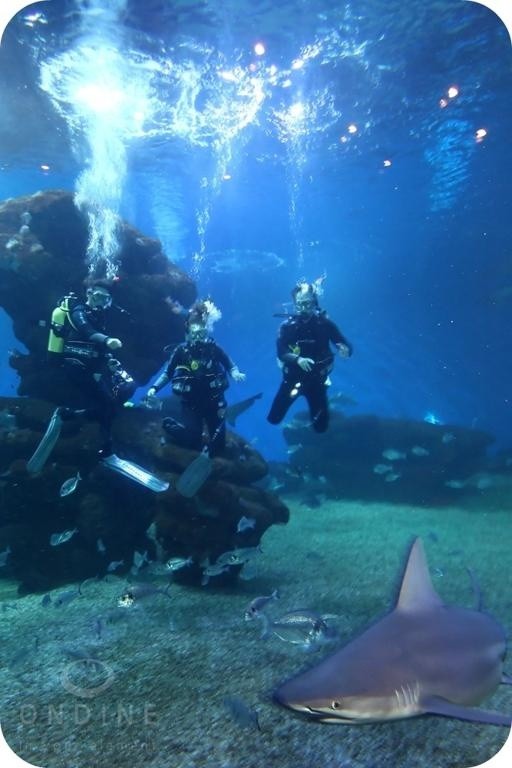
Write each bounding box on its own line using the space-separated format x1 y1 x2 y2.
295 300 312 312
190 329 207 339
92 291 111 303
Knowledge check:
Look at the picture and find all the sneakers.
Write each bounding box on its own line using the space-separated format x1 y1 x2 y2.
97 450 119 466
56 407 69 430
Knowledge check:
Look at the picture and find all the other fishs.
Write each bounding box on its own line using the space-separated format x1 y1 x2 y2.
41 392 503 655
0 547 12 567
0 602 18 613
220 696 261 732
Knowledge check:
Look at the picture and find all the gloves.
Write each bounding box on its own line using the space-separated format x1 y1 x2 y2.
340 345 349 357
231 370 246 382
106 338 122 350
297 357 315 372
148 388 156 396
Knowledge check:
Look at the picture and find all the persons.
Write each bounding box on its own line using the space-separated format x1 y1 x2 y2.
61 282 122 456
148 309 246 451
267 283 352 433
